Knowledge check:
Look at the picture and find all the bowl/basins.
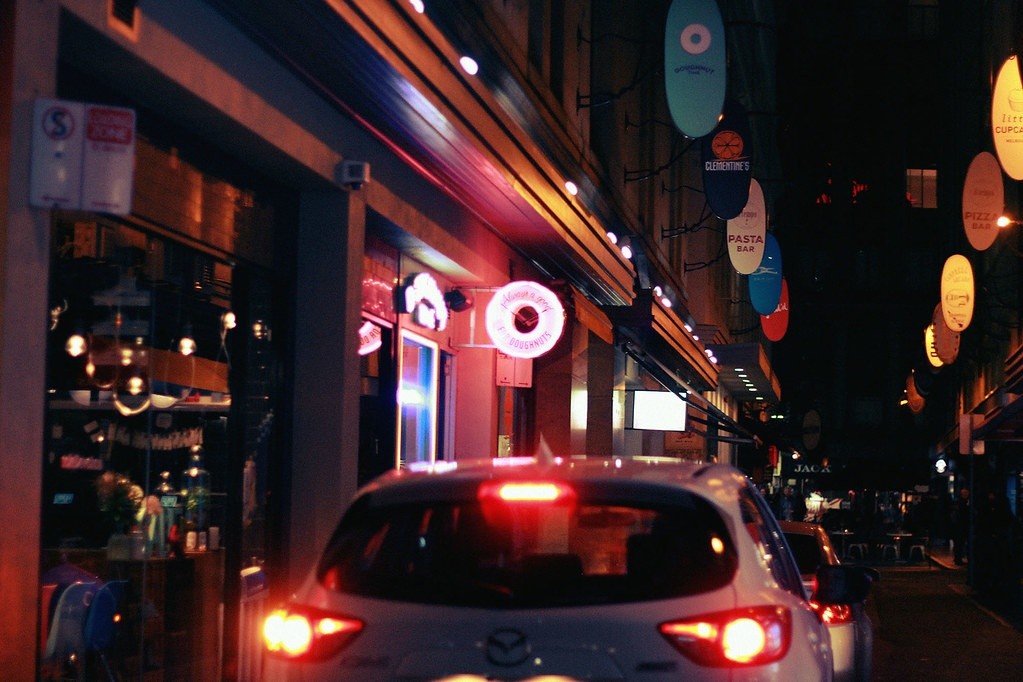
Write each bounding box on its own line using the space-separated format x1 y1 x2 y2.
69 390 112 405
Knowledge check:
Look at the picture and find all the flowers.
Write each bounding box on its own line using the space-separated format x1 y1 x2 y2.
93 470 143 533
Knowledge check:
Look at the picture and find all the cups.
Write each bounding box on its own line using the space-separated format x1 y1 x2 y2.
209 527 219 551
186 532 197 549
199 531 206 552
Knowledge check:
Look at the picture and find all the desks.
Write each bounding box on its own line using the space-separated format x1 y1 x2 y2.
887 533 913 562
833 529 855 561
43 546 226 682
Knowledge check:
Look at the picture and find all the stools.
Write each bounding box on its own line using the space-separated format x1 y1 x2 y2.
882 544 898 561
909 544 926 562
848 544 865 562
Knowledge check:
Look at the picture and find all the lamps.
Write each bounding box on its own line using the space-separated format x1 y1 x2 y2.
343 160 371 190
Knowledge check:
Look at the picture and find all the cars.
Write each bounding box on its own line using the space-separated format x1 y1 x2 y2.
257 457 873 682
774 521 876 682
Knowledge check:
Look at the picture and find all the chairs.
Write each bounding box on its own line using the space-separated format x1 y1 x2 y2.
44 580 128 682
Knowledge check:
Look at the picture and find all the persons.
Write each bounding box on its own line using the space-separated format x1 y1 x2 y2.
645 505 717 580
467 497 514 569
988 494 1023 611
760 484 831 523
951 488 971 566
93 470 145 550
37 533 117 682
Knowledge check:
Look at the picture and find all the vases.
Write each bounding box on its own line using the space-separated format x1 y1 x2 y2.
105 530 130 561
